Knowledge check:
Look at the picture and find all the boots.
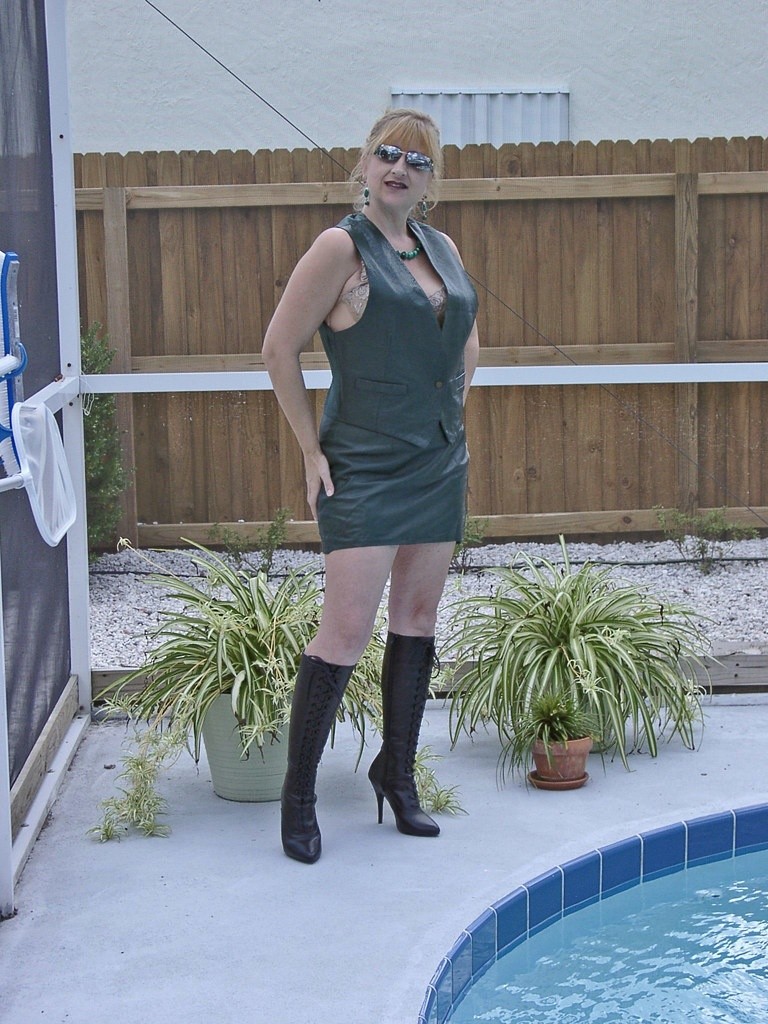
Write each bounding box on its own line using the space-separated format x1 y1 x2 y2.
280 653 358 864
366 628 439 837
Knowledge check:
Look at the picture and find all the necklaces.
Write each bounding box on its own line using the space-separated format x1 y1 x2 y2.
393 231 421 262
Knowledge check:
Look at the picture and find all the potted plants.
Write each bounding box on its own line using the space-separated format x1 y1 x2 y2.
445 533 703 770
91 538 466 843
497 695 601 790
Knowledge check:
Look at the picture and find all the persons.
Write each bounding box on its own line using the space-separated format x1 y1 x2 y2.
260 106 480 865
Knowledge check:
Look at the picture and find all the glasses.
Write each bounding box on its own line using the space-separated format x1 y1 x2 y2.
373 143 433 173
